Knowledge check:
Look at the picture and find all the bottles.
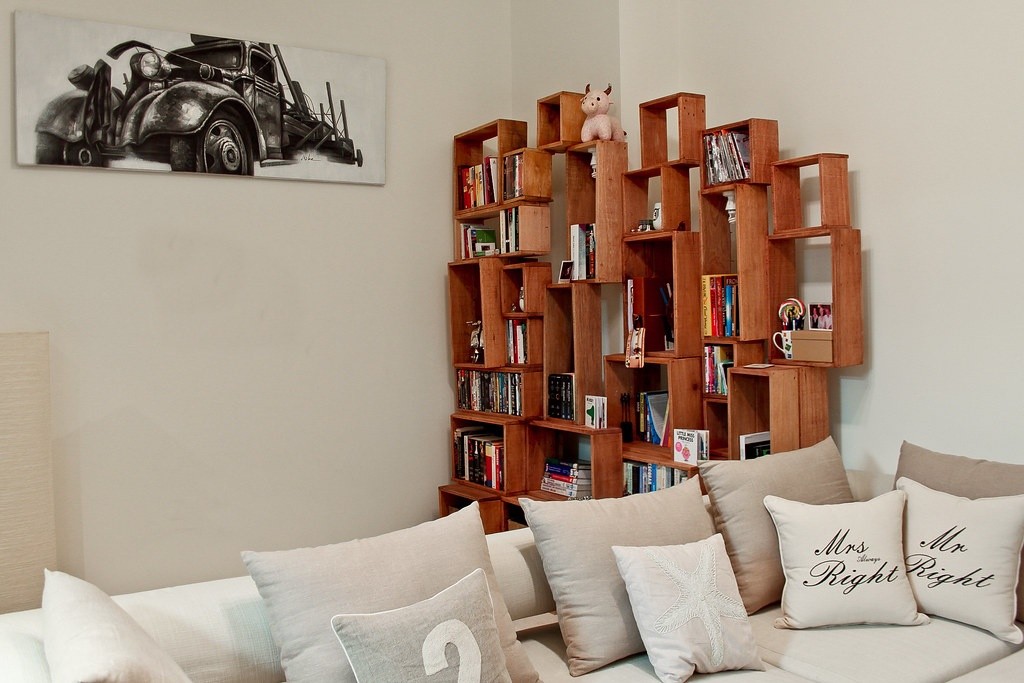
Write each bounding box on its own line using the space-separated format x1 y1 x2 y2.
653 202 661 229
519 287 524 312
638 220 653 230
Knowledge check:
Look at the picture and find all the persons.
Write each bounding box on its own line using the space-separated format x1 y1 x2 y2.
810 308 831 329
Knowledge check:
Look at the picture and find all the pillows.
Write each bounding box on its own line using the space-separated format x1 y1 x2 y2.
329 567 512 683
611 532 767 683
41 567 192 683
516 473 714 676
894 477 1024 645
238 500 544 683
892 440 1024 622
695 435 855 615
762 490 932 629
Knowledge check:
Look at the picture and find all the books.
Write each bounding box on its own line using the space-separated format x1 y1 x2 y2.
624 462 686 494
457 370 522 417
503 153 522 200
673 429 710 465
453 426 504 491
702 274 739 337
506 319 528 363
703 132 750 184
458 155 498 210
585 396 607 430
740 431 770 460
540 457 591 498
461 206 519 259
548 373 575 420
636 390 669 447
704 345 733 396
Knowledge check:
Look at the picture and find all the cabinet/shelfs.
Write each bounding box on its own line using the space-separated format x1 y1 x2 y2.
438 90 864 534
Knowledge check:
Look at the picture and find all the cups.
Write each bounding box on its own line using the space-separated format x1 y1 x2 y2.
772 330 792 360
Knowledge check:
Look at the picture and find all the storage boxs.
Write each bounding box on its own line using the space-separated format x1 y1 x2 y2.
790 330 834 363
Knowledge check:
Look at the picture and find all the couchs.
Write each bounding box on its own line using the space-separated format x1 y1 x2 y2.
0 469 1024 683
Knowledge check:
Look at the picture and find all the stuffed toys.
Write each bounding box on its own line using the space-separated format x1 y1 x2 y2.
580 82 626 143
